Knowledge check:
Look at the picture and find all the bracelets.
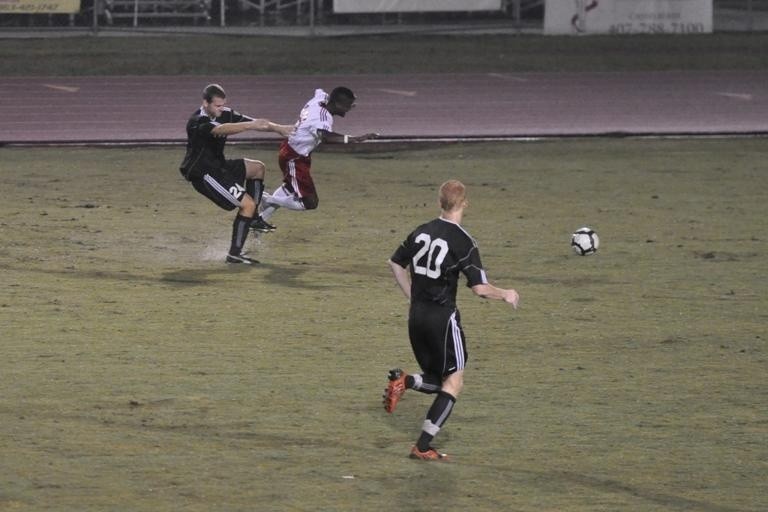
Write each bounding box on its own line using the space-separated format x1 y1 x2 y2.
344 134 349 144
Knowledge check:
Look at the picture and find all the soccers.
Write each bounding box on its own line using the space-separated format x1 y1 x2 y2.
571 227 599 255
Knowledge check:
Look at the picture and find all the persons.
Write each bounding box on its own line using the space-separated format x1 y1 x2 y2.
178 83 298 264
251 84 357 244
381 178 520 461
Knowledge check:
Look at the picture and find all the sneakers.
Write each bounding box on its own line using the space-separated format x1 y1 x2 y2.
382 368 408 413
256 192 271 214
224 253 258 264
409 444 448 461
248 215 277 233
